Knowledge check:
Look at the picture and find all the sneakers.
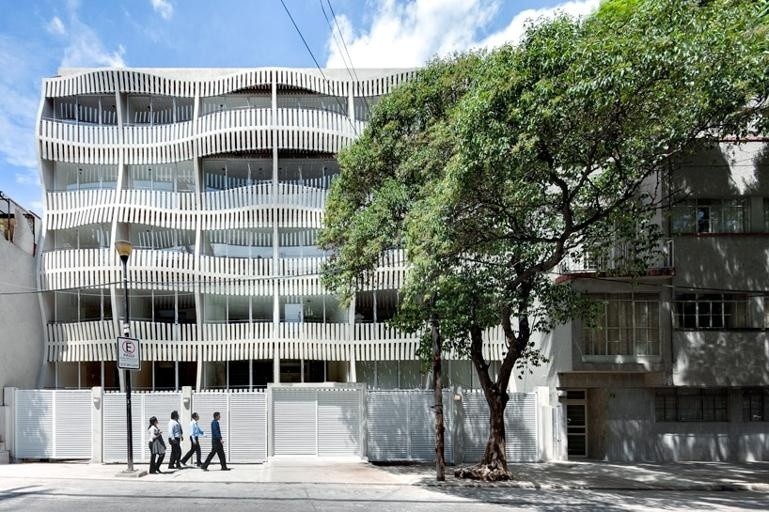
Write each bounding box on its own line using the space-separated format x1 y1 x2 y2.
169 461 186 469
197 462 208 471
222 468 230 470
150 468 161 474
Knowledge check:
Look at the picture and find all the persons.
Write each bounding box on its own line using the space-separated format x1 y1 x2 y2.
201 412 231 472
180 412 207 467
168 410 184 470
147 415 166 475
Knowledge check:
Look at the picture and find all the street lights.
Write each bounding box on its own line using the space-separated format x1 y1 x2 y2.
112 237 135 473
